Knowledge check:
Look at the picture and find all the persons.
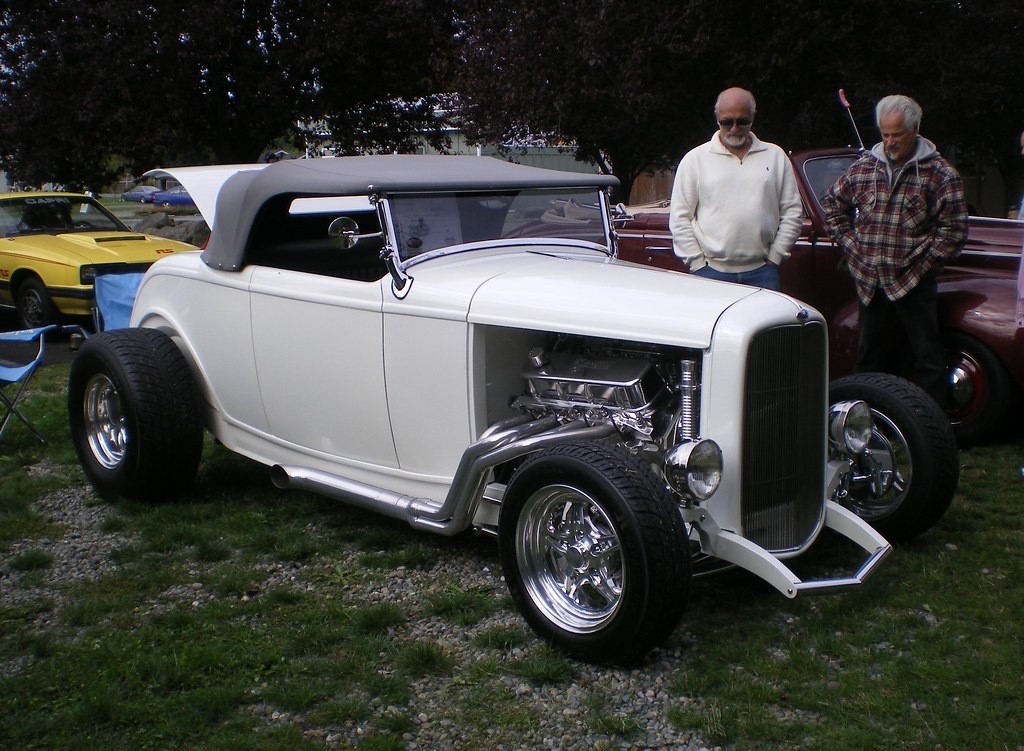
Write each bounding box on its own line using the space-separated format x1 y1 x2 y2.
1007 131 1024 219
823 95 969 384
669 87 803 291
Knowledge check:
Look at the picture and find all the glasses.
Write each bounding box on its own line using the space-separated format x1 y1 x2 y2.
716 112 752 128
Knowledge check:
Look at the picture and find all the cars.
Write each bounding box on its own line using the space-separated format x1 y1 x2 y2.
503 148 1024 443
153 185 195 207
121 186 161 203
70 154 960 665
0 191 200 337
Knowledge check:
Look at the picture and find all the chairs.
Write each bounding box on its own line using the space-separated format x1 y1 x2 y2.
0 303 57 446
15 204 62 231
62 268 146 352
